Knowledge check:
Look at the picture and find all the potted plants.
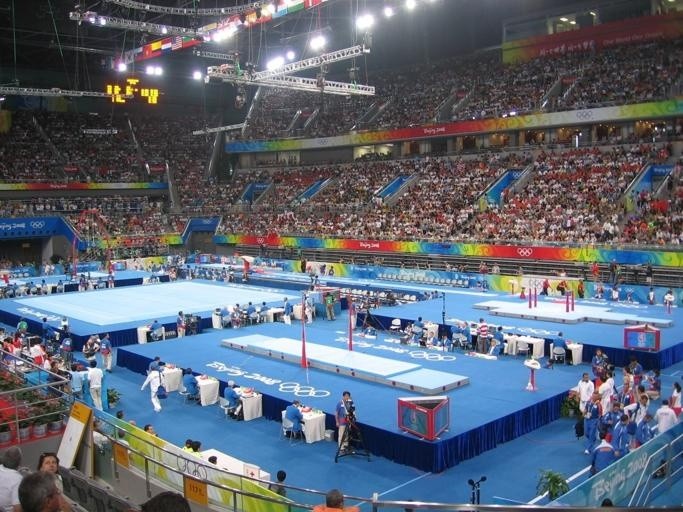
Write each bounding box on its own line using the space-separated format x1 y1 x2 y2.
106 387 123 408
559 394 579 416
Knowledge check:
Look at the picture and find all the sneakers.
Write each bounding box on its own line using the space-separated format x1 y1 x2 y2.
347 448 354 454
106 369 112 373
339 450 345 455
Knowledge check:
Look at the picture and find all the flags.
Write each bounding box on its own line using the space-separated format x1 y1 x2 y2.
160 0 327 58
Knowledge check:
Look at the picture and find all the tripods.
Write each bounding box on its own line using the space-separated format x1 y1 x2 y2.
335 416 372 464
362 310 380 333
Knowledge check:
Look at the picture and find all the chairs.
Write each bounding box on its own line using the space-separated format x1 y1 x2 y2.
278 417 302 445
16 465 142 512
388 319 401 332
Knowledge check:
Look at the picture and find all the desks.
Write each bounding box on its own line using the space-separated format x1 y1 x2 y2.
156 365 262 422
281 406 325 443
411 322 583 366
136 325 165 344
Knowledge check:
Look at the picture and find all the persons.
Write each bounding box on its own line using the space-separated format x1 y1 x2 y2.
575 349 680 478
140 365 164 412
224 380 242 419
284 399 303 440
313 489 360 512
148 292 335 342
552 332 567 363
1 37 682 307
0 411 286 511
148 357 163 373
0 317 112 411
336 390 354 455
400 316 507 356
182 368 199 401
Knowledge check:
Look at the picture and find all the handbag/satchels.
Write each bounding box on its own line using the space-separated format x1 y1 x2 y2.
157 386 167 399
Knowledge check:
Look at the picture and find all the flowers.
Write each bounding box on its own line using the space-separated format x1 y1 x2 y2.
0 370 69 432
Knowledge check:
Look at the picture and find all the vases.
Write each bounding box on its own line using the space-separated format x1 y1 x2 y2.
0 419 63 445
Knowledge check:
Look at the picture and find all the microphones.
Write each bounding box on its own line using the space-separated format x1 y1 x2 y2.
467 479 478 489
475 476 487 484
